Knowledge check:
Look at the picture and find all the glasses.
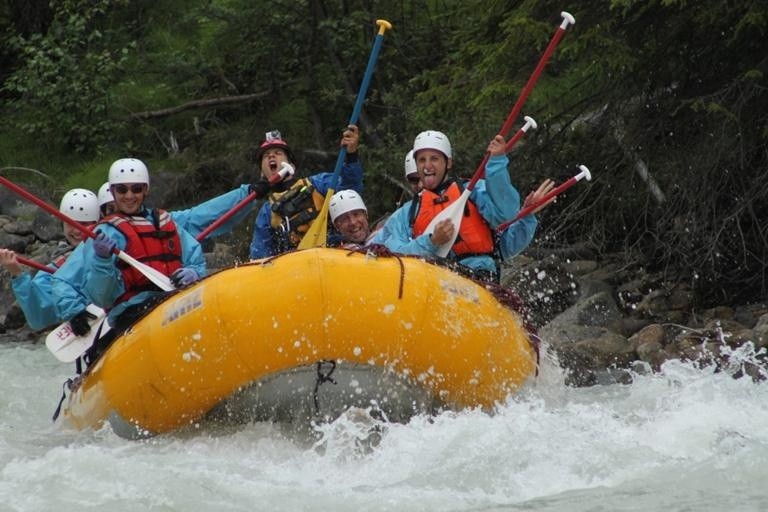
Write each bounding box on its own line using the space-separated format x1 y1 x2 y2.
111 184 144 194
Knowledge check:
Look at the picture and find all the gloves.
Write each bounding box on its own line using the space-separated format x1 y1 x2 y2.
170 266 201 289
249 175 272 199
93 228 117 259
69 308 98 336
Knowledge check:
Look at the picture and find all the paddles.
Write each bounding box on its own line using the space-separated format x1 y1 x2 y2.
46 162 295 364
424 11 575 260
295 20 392 249
0 175 176 292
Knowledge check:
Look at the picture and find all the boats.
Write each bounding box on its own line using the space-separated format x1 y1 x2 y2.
60 247 541 439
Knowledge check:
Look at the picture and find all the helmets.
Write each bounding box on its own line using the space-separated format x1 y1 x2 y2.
412 129 454 160
255 137 296 158
108 157 151 192
59 188 101 225
327 189 368 226
97 182 116 207
404 149 418 178
